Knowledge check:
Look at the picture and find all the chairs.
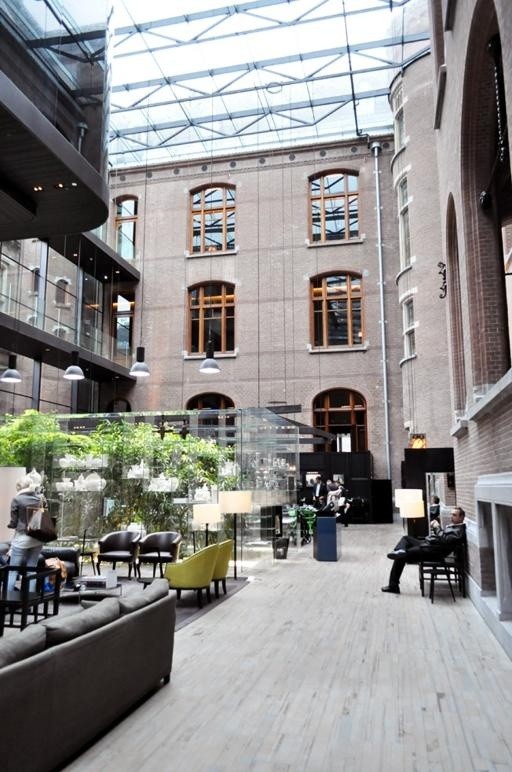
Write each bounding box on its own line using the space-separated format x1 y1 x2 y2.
0 530 235 635
417 541 466 604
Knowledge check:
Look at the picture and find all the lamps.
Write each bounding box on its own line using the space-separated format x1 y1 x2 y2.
0 241 22 382
129 80 150 377
412 435 425 449
64 234 85 381
194 504 220 546
219 490 252 579
200 69 220 373
395 489 426 564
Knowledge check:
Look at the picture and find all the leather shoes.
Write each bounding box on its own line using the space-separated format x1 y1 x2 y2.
382 586 400 593
388 549 407 560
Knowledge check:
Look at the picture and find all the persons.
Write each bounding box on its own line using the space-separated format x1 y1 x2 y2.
7 479 48 612
381 507 466 593
296 476 353 524
430 495 440 521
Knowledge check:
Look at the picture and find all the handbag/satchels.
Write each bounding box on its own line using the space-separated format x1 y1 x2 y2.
26 509 57 542
425 533 441 545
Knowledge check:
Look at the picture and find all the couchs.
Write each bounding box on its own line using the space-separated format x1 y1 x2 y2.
0 577 176 772
